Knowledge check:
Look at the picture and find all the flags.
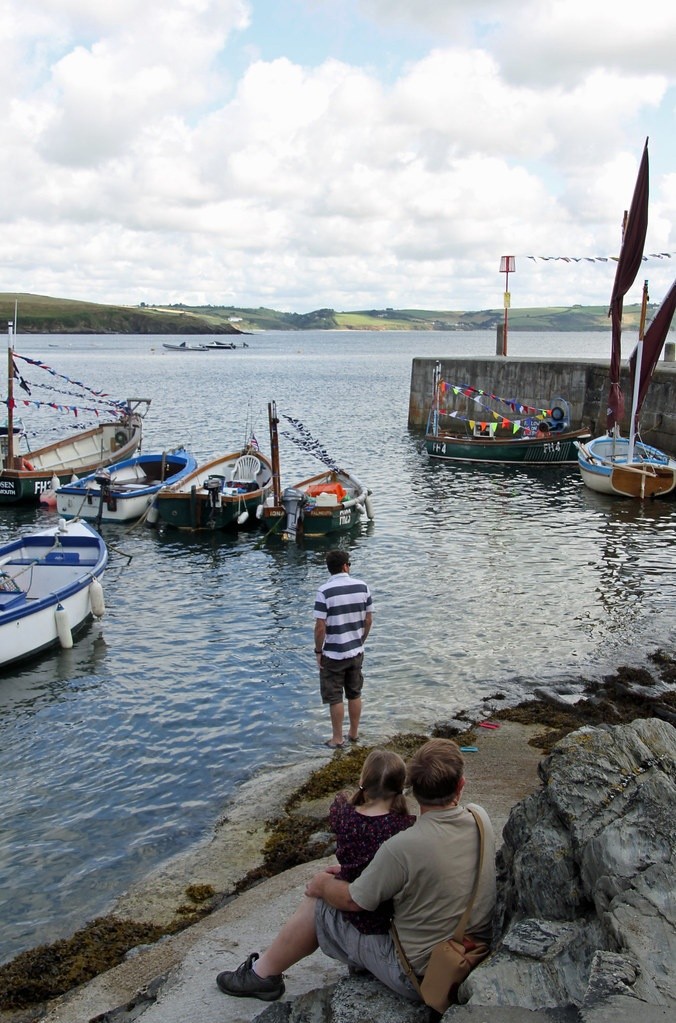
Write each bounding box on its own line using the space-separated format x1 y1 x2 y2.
523 252 673 262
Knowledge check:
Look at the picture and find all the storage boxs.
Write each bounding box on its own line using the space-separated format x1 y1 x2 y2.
304 484 354 507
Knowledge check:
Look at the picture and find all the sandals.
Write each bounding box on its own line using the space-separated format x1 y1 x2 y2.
322 740 345 750
344 735 360 742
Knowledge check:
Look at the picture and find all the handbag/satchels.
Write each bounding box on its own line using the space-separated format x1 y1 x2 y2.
420 934 492 1016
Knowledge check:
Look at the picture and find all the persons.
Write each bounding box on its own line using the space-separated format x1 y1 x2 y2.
216 738 495 1002
313 551 372 744
328 749 417 936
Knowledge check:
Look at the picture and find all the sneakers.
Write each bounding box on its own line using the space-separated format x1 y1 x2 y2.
216 952 286 1002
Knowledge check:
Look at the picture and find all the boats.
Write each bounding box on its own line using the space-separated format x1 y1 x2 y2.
55 444 199 525
143 399 275 533
0 515 110 669
423 358 591 464
261 400 376 541
163 339 236 351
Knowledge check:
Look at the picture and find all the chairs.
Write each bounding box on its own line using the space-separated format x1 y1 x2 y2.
231 455 260 483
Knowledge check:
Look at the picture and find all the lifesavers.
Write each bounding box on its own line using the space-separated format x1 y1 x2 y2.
551 406 564 420
21 457 35 471
115 430 127 443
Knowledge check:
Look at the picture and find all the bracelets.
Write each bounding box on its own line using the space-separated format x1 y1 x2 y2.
314 648 322 654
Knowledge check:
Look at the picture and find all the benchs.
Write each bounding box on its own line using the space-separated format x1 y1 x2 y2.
111 478 163 492
341 487 357 491
5 552 97 567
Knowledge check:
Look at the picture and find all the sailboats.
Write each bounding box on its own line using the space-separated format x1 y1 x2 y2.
0 300 143 506
572 278 676 499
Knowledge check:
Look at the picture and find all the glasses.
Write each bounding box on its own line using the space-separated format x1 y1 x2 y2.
343 563 351 566
